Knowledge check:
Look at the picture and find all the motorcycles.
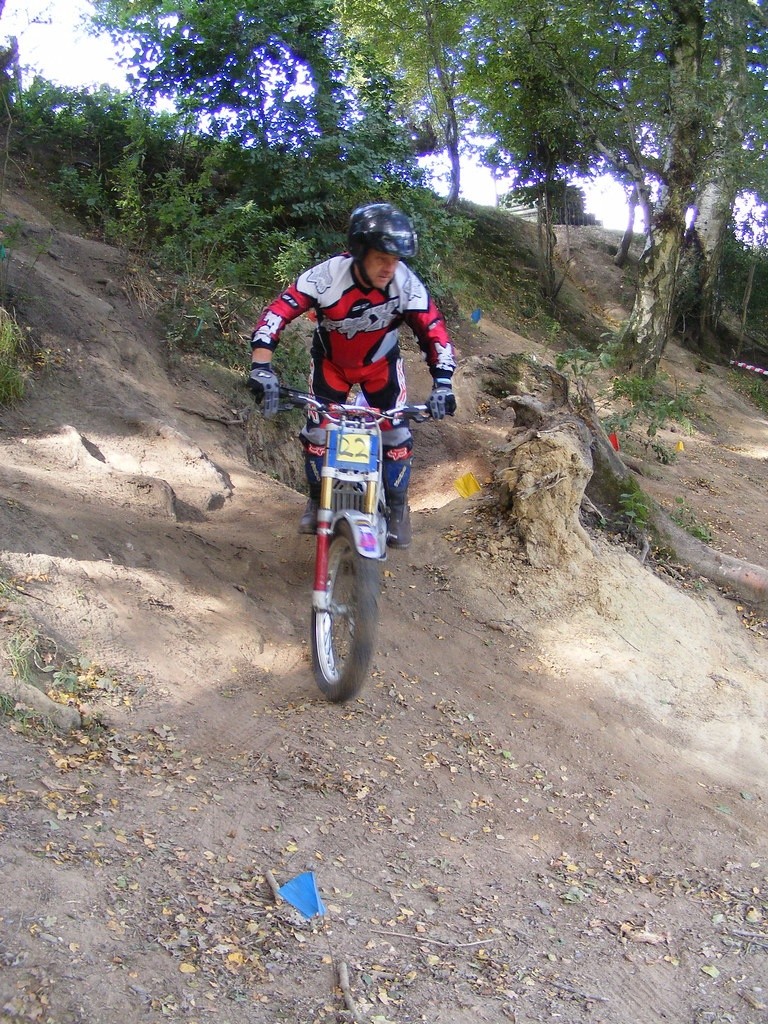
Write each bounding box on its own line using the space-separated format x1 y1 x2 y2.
239 381 456 706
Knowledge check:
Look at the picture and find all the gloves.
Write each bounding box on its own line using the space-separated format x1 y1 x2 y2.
422 379 456 421
249 361 280 419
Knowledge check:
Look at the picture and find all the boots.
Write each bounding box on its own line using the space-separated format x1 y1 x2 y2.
299 481 324 534
383 487 411 548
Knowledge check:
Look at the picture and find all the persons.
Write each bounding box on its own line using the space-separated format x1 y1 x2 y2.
247 204 458 548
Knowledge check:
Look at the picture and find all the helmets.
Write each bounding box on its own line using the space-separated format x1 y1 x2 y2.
348 203 418 258
